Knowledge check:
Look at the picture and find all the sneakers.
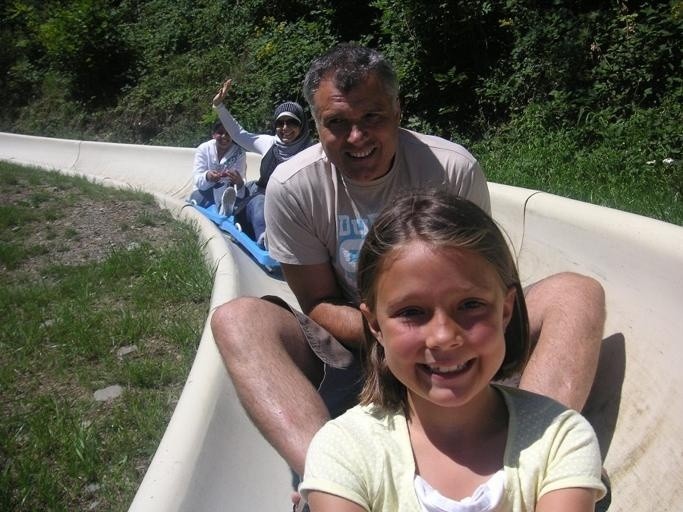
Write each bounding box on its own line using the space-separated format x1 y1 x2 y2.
219 187 236 216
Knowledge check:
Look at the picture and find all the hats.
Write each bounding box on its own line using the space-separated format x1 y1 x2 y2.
275 103 302 125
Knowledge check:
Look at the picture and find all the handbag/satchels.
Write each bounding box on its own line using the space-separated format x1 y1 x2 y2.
234 180 258 231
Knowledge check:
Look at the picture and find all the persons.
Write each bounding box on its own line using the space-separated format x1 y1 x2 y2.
208 41 613 511
296 185 609 511
211 77 316 252
187 119 249 220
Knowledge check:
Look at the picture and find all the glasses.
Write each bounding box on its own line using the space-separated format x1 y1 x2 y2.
276 119 299 128
